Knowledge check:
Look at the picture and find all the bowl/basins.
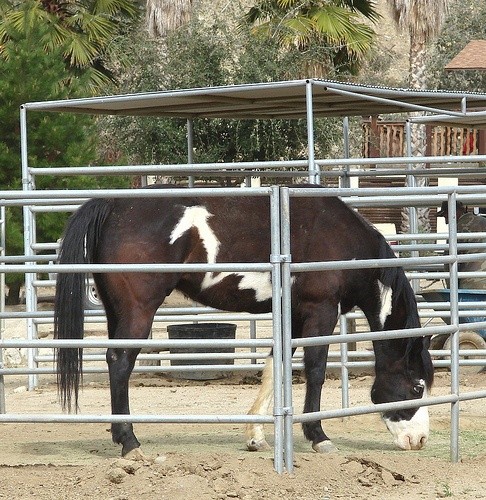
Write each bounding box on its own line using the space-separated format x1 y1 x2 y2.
167 323 237 380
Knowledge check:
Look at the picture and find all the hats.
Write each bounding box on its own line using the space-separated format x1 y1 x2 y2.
436 200 463 217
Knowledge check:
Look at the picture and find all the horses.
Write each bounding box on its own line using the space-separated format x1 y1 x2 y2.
53 183 435 462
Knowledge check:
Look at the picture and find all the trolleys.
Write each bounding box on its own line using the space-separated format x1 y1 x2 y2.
415 289 486 373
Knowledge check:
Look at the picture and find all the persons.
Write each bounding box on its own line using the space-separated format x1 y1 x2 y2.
437 200 486 289
